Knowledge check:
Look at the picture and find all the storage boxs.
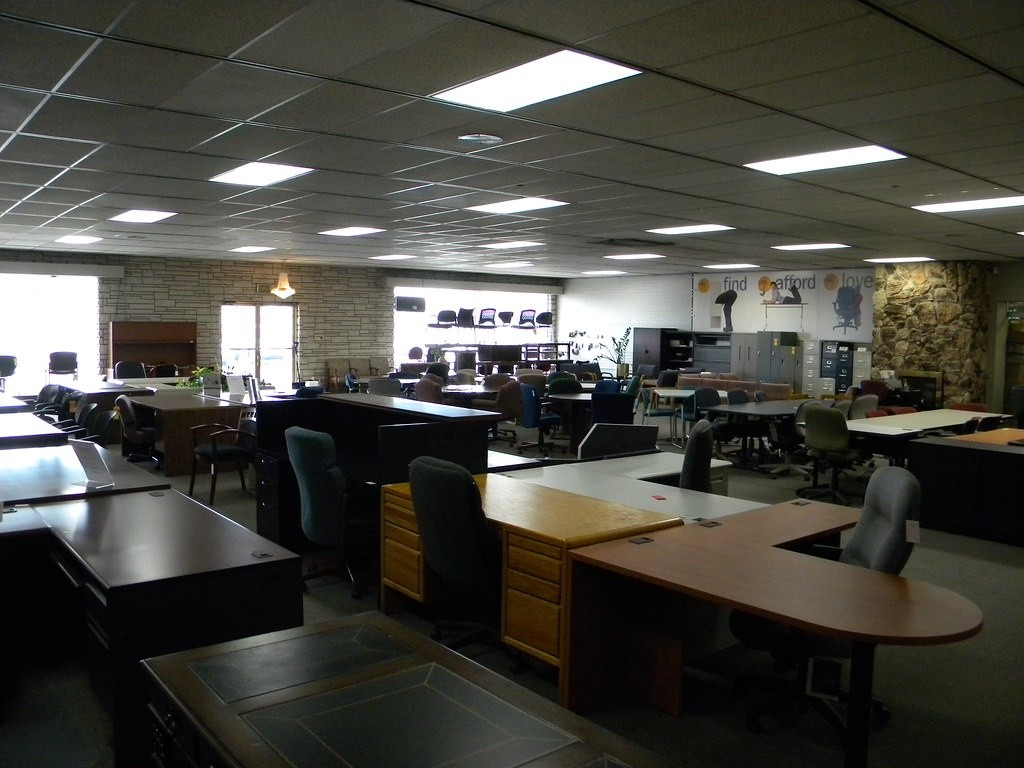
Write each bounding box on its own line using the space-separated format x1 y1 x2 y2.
801 339 872 399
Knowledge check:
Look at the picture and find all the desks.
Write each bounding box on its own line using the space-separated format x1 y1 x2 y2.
355 378 419 386
548 395 594 404
0 382 984 768
544 382 595 392
474 376 516 382
696 398 839 418
655 387 728 401
441 384 498 399
419 372 459 378
801 409 1010 442
328 344 1014 514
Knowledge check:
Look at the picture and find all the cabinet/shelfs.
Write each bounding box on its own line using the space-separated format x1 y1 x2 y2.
780 345 801 394
693 332 732 374
665 330 695 368
632 326 676 380
730 333 757 379
756 329 797 384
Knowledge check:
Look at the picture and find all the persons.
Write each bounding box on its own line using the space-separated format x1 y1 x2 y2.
763 282 802 304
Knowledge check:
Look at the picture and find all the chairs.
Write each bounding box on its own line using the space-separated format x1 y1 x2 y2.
803 465 925 578
405 457 499 657
284 425 379 598
662 420 717 494
0 353 275 504
